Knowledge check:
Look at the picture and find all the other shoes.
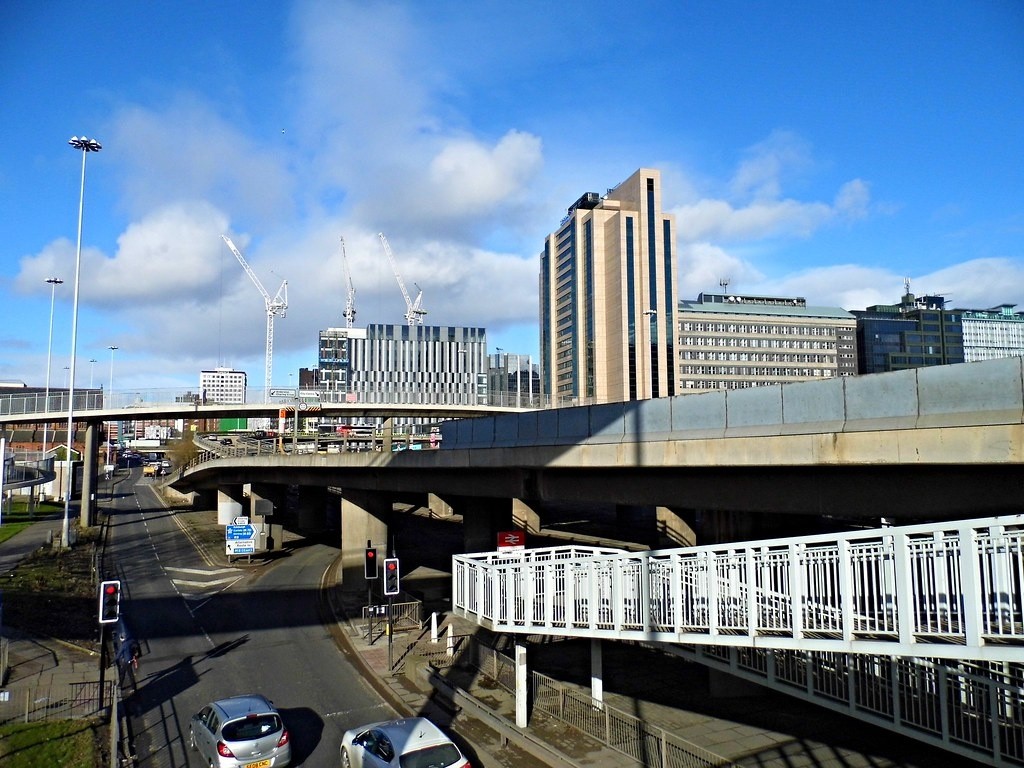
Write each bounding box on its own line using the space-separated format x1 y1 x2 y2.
114 685 122 688
129 690 137 693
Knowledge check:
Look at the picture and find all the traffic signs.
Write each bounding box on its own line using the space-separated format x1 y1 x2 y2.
226 540 255 555
226 524 259 539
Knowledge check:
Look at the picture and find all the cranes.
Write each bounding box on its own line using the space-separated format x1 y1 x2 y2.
221 234 289 404
379 232 428 326
339 234 356 330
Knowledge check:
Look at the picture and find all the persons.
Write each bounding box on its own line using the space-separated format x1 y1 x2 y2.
111 634 137 693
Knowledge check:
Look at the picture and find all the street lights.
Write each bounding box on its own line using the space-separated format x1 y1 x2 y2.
63 367 69 389
43 277 64 462
108 345 118 409
61 134 103 547
91 360 98 389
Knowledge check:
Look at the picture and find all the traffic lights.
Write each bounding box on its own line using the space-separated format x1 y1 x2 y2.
386 561 397 592
366 548 376 577
102 582 117 620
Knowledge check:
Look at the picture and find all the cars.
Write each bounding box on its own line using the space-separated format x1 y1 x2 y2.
221 439 232 446
209 434 217 441
189 692 291 768
340 717 472 768
